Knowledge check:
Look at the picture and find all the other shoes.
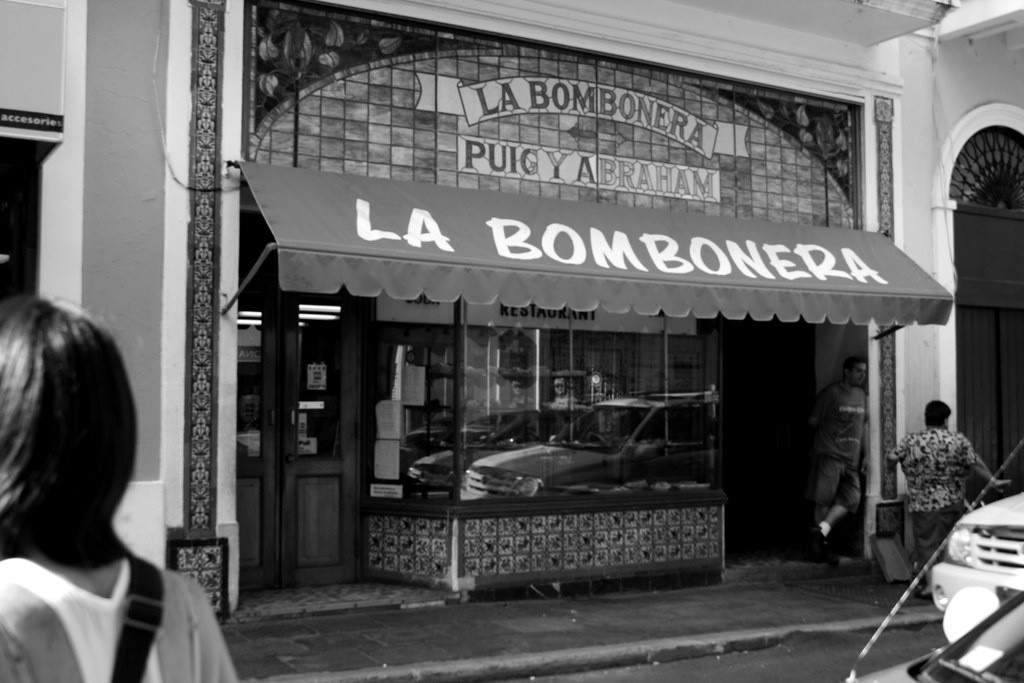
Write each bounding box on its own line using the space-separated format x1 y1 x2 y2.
810 526 841 566
913 570 932 596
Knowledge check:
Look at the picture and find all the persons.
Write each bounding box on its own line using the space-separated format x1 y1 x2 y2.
806 355 869 567
551 378 578 405
886 400 1012 598
0 293 241 683
509 380 534 406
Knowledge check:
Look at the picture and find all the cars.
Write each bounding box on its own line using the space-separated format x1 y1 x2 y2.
850 593 1024 682
932 491 1024 617
402 404 592 499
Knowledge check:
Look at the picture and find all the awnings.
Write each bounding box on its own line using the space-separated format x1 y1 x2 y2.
221 160 953 339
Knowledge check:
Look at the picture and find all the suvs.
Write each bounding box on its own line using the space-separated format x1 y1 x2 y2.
458 390 714 505
